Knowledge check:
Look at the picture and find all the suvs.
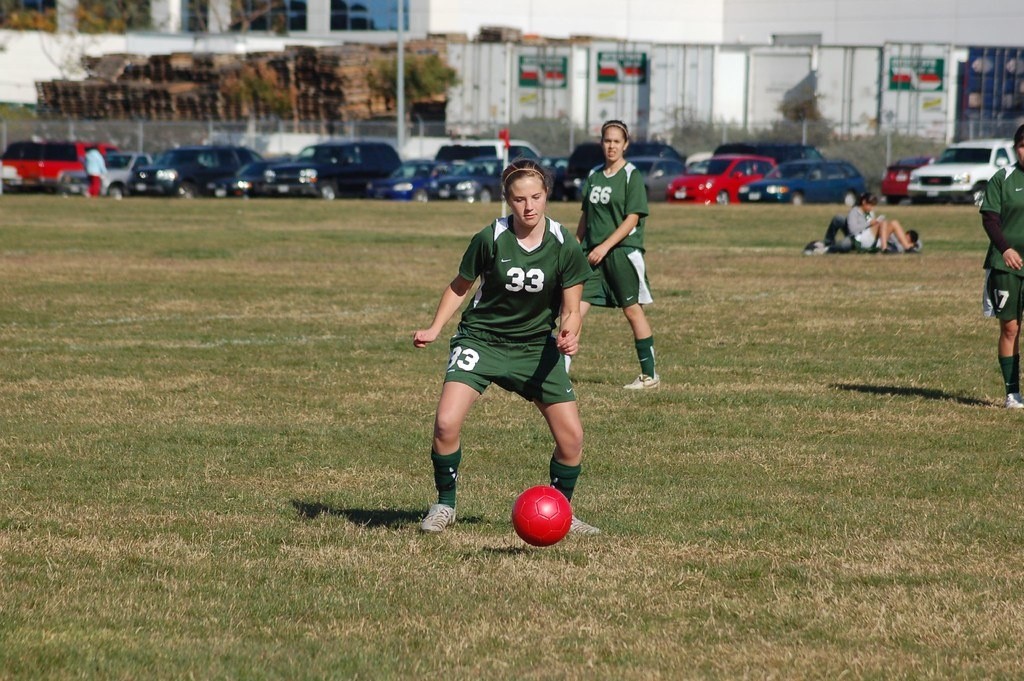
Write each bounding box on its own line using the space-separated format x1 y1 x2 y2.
907 136 1017 207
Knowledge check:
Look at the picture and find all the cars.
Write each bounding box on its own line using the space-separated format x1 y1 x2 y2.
881 156 938 204
737 158 867 210
0 134 826 208
666 153 783 207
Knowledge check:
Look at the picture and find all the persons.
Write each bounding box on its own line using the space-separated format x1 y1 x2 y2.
566 119 662 389
85 143 109 197
412 160 603 534
979 122 1024 408
813 192 921 256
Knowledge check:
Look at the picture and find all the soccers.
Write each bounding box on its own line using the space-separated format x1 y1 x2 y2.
512 485 573 547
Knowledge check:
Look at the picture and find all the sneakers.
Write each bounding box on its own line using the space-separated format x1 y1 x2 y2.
623 374 660 391
1006 393 1024 408
420 502 457 532
569 514 601 534
905 246 920 253
882 248 893 254
